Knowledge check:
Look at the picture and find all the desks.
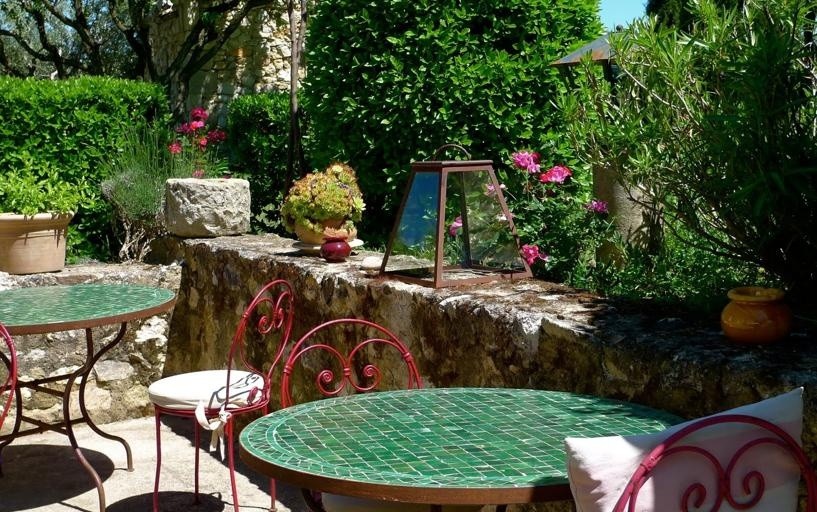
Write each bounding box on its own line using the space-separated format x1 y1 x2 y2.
0 282 177 511
237 386 693 511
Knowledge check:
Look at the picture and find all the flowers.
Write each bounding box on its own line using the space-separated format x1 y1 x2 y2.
452 148 613 281
166 105 230 178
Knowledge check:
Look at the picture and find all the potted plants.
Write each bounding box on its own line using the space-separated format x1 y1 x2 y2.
1 157 98 273
279 159 368 245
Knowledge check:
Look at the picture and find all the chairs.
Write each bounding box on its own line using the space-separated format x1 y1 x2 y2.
148 280 294 510
608 411 816 512
281 317 421 511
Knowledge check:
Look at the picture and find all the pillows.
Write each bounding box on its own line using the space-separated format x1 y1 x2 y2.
562 386 806 512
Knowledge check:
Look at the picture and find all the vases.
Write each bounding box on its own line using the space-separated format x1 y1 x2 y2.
321 236 352 262
722 284 793 346
